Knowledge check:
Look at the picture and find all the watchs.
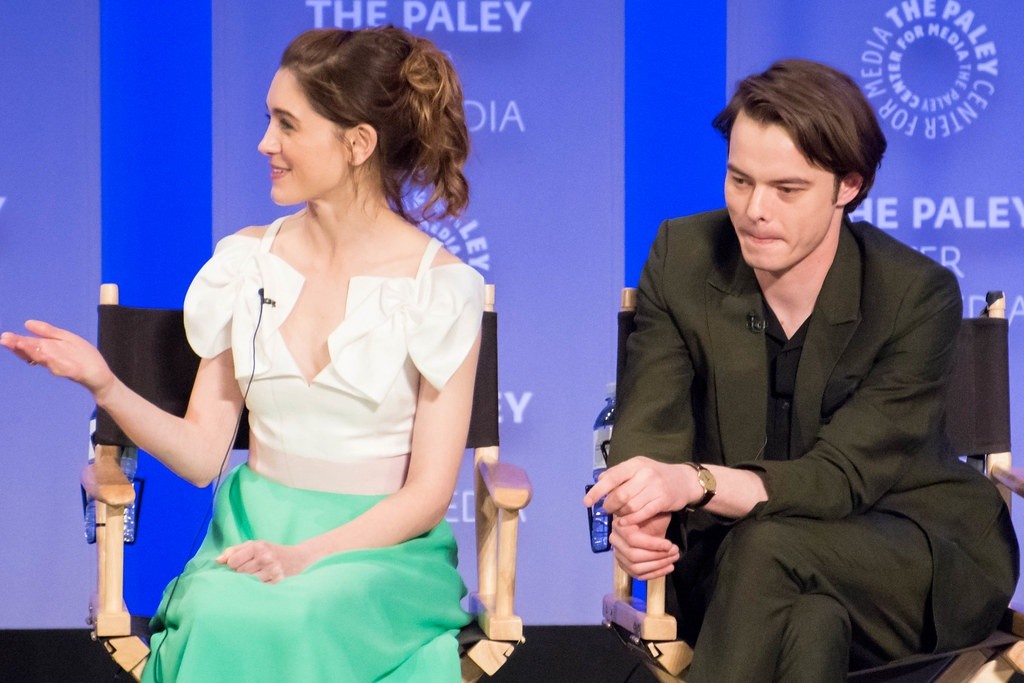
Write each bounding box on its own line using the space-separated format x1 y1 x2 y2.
682 462 716 512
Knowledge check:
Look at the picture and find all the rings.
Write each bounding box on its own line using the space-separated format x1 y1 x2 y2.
29 348 40 365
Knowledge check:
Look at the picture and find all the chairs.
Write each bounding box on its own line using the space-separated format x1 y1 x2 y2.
82 284 531 683
602 287 1024 682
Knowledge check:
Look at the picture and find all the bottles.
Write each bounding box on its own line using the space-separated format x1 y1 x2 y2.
85 404 137 544
593 383 615 553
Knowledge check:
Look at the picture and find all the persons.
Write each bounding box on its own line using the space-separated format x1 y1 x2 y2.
0 27 486 683
583 60 1020 683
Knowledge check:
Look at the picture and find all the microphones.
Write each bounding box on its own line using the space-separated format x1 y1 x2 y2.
258 289 264 303
747 310 765 333
